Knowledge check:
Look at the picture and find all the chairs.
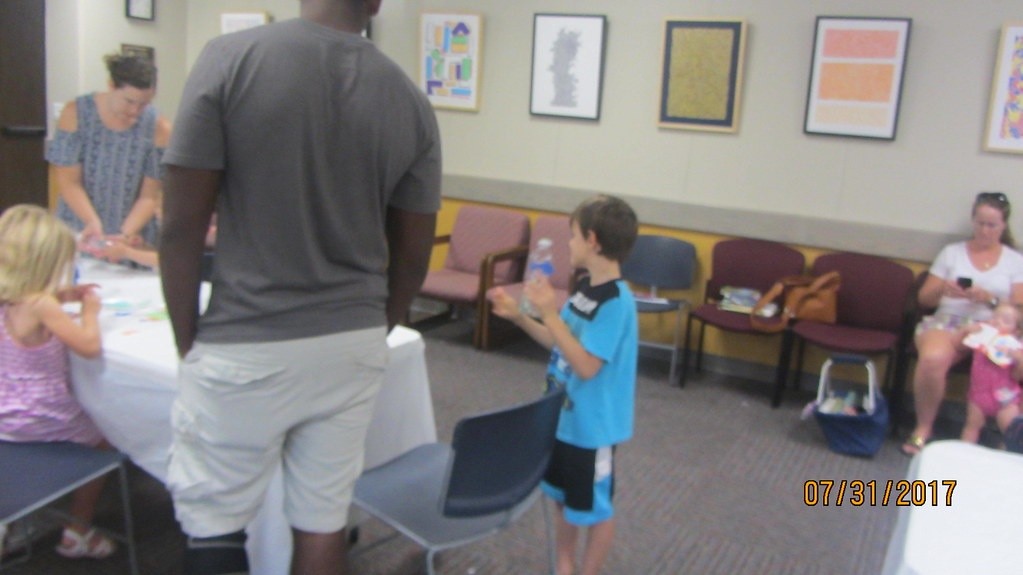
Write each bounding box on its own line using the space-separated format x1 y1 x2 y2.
353 383 573 575
402 207 973 438
0 439 139 575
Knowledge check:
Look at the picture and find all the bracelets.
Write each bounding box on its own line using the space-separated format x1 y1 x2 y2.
988 297 999 309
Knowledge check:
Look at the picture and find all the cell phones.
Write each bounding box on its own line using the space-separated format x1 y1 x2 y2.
958 278 972 290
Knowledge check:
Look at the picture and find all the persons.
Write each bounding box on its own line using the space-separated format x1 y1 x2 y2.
81 210 218 282
903 191 1023 455
45 52 171 271
960 305 1023 444
488 194 638 575
161 0 442 575
0 203 118 561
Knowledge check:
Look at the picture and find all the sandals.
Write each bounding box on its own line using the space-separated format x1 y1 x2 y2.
57 527 118 557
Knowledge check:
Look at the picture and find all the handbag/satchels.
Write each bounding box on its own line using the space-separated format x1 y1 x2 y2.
750 270 842 333
817 353 890 456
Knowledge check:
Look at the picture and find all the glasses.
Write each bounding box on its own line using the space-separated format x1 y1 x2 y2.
978 193 1007 207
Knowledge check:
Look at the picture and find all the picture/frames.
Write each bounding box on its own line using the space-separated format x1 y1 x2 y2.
982 21 1023 154
657 16 746 134
125 0 154 21
529 13 606 120
415 8 484 111
218 8 268 34
803 17 910 140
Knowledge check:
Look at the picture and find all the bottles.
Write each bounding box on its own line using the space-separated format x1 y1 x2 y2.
819 389 858 416
520 239 555 319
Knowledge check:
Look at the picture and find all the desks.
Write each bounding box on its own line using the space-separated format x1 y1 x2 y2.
67 256 437 575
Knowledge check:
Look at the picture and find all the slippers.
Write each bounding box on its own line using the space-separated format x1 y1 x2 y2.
903 431 928 458
